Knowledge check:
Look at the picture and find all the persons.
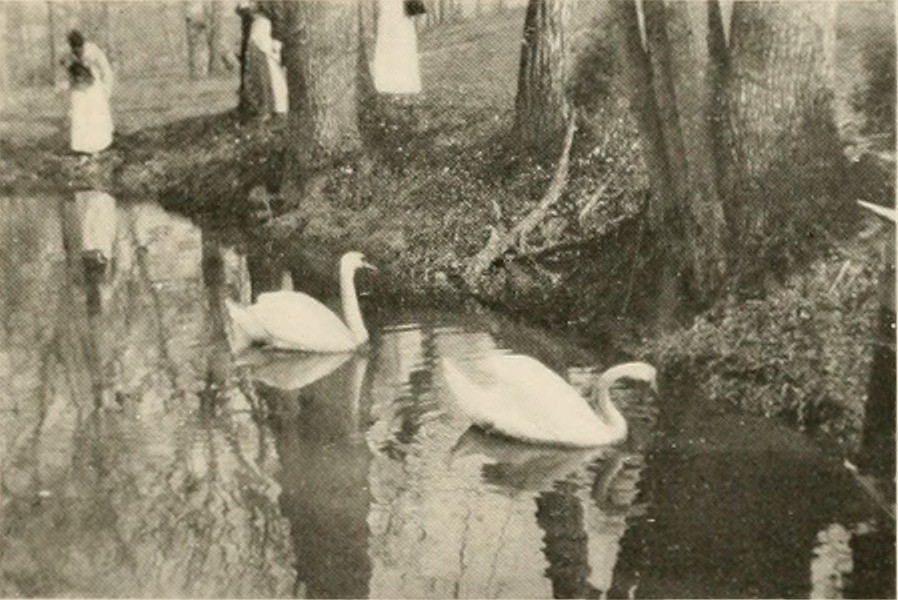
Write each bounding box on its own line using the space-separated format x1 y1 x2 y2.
55 29 118 155
236 2 289 127
372 0 422 94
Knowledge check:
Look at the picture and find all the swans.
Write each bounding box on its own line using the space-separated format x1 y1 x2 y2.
225 252 379 351
442 354 659 446
